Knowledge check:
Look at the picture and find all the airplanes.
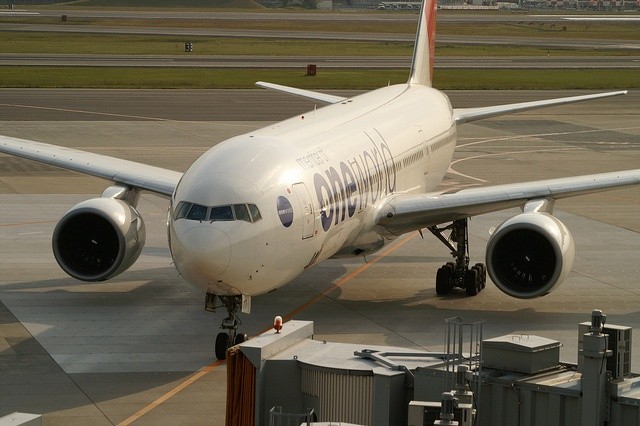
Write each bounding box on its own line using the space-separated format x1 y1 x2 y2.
0 0 640 361
